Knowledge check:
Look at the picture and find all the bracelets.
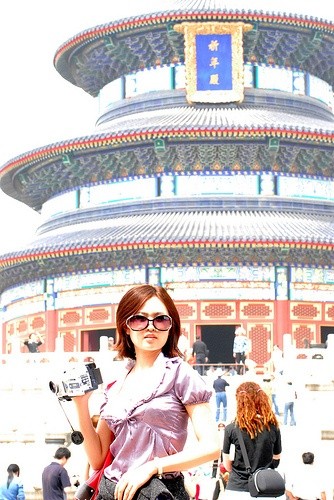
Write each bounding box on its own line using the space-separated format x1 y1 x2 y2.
155 456 162 478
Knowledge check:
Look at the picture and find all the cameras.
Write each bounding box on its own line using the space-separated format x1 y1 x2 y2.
48 361 103 401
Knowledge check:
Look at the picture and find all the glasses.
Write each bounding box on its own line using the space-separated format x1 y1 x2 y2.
126 314 172 332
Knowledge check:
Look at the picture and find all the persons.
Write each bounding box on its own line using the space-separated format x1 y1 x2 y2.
272 345 284 374
0 464 25 500
262 378 280 416
233 328 249 375
213 373 230 423
284 451 326 500
220 381 286 500
42 447 71 500
107 337 114 351
283 382 298 425
191 335 208 376
20 329 43 353
66 285 221 500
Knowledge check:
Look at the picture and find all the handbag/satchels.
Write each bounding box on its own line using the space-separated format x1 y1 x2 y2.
248 467 285 497
